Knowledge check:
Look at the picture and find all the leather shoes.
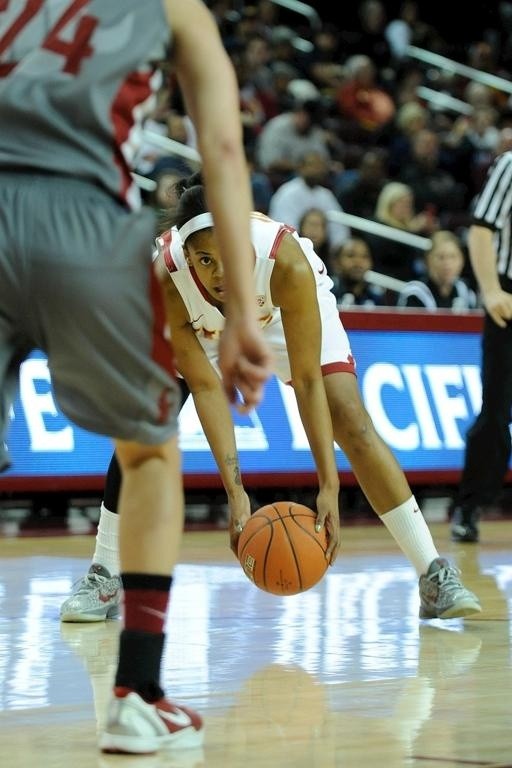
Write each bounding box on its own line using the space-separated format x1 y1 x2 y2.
448 498 480 544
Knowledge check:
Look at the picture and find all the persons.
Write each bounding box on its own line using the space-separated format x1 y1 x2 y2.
58 174 487 628
131 0 512 309
441 152 511 549
1 1 276 753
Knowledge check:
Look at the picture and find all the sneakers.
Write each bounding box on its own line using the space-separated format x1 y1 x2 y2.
58 562 123 623
417 559 483 622
97 683 208 759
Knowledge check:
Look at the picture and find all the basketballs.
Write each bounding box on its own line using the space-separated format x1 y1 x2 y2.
239 500 331 597
